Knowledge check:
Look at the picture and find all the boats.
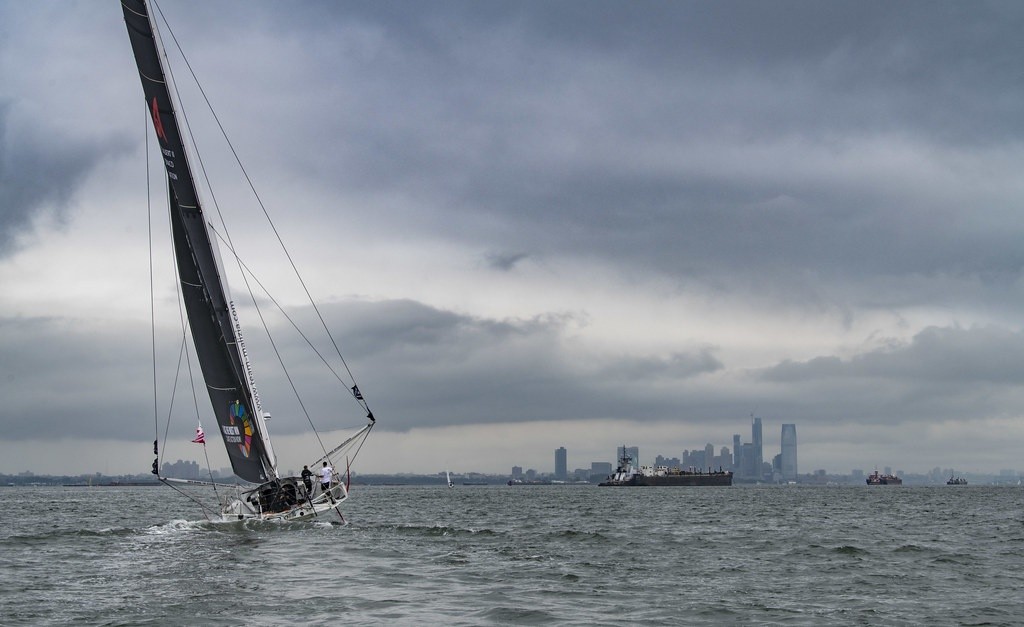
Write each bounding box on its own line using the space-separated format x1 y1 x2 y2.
597 444 734 487
946 475 968 485
865 469 903 486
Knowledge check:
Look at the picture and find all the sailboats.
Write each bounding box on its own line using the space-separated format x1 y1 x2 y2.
119 0 376 530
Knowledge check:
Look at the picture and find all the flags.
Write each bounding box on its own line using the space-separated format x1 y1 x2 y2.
192 429 205 444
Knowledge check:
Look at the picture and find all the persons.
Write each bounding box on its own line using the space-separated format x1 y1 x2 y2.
301 465 313 491
319 461 336 504
